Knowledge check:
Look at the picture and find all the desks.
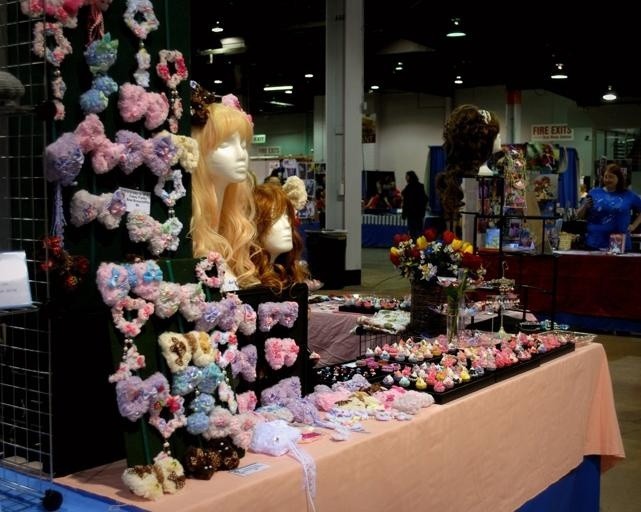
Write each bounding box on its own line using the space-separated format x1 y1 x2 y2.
58 340 606 512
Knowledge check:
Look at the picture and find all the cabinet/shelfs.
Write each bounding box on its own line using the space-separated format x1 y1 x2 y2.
473 243 641 331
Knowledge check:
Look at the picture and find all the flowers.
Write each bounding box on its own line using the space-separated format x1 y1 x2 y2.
387 227 472 288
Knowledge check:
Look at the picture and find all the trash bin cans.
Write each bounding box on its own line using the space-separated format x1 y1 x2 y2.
305 229 348 290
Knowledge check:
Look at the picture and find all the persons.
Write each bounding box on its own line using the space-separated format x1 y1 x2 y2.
360 180 404 213
442 102 506 179
182 103 261 290
248 178 309 289
401 170 430 240
577 163 641 253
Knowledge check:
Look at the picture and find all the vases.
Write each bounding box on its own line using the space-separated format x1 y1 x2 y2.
406 279 471 342
